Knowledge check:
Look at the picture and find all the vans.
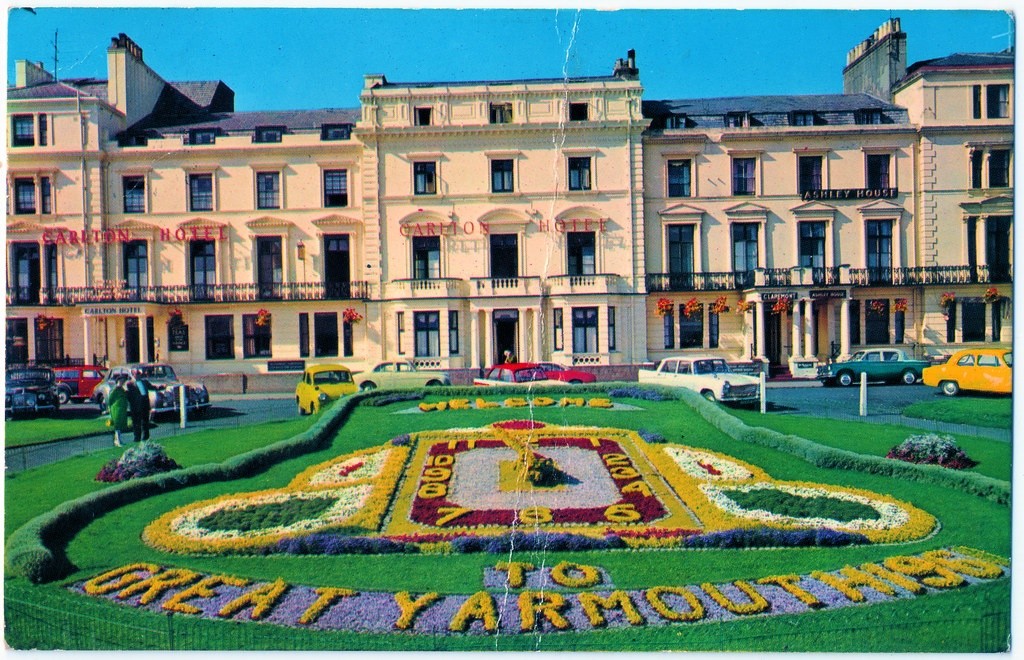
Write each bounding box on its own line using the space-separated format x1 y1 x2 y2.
51 365 109 404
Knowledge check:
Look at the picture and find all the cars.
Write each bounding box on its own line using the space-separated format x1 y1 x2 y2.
817 347 930 387
535 362 597 384
922 348 1012 395
92 361 211 422
473 363 569 388
5 368 59 420
296 364 358 416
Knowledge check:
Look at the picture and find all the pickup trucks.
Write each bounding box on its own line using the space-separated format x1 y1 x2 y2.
352 360 450 393
638 356 762 405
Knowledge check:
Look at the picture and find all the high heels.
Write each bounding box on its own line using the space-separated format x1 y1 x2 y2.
114 440 125 448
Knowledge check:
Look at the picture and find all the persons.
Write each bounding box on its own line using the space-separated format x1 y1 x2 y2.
105 368 168 448
504 349 517 363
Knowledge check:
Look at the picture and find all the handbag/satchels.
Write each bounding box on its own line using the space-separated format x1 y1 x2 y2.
106 420 113 427
